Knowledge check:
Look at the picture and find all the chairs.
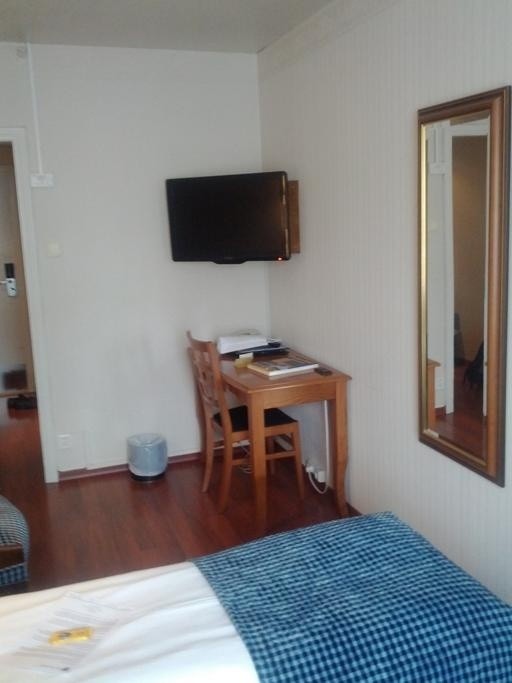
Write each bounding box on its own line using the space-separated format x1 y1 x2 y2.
0 493 32 598
187 330 306 513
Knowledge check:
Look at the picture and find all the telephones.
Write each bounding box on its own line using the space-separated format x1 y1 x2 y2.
237 328 263 335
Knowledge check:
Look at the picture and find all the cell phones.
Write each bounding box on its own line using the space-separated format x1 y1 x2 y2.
315 366 331 376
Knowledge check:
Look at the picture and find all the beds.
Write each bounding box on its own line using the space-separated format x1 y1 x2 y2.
1 512 512 683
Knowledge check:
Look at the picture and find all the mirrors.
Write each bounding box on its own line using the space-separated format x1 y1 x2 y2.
417 85 511 489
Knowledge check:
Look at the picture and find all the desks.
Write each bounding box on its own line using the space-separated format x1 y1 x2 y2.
427 360 440 433
190 349 353 528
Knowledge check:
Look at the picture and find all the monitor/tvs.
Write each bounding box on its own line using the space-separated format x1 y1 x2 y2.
165 171 291 264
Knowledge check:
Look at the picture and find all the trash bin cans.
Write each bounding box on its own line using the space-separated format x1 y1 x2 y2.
127 433 166 481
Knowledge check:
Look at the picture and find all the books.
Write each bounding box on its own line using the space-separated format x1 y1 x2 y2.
249 354 319 381
249 369 315 381
